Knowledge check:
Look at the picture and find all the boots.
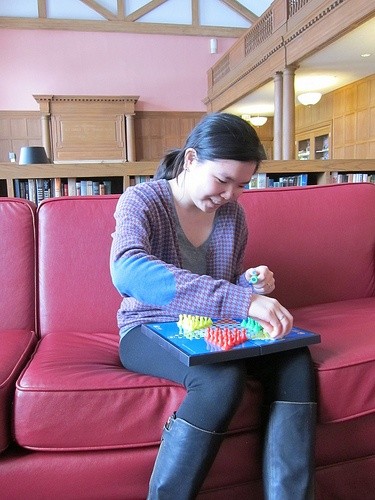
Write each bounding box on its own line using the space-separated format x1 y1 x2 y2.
261 401 318 500
147 411 227 500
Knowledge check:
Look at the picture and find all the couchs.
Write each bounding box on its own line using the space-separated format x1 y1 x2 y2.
0 182 375 500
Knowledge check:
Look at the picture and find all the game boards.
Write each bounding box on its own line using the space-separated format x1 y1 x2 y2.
141 318 321 368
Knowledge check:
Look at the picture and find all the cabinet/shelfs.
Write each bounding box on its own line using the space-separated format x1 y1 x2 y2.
295 124 330 161
0 159 375 205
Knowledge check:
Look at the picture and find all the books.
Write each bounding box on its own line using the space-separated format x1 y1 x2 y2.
14 175 153 205
242 172 375 188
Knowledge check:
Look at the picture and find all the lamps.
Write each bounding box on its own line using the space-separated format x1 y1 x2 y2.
20 146 49 206
297 92 322 109
250 113 267 129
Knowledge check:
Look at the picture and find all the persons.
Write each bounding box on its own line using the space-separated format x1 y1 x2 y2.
108 113 318 500
